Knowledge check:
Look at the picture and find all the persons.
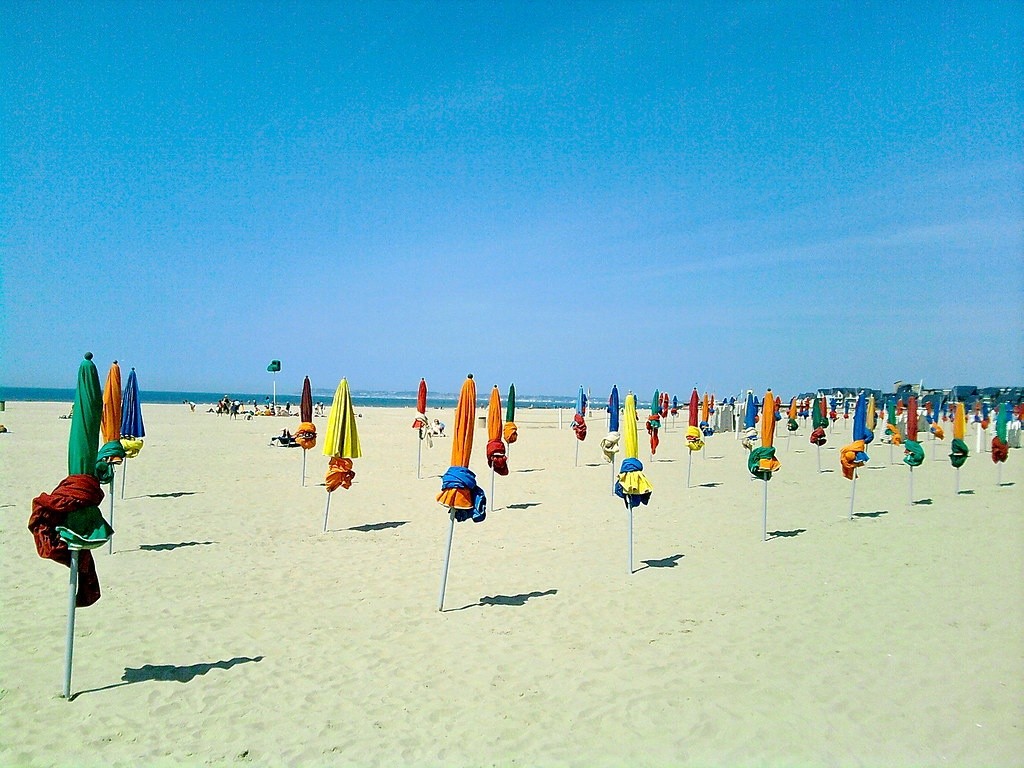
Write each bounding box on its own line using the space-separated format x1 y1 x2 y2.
314 401 326 415
189 401 196 412
278 426 298 443
286 401 290 411
434 419 445 437
206 395 274 421
66 405 74 418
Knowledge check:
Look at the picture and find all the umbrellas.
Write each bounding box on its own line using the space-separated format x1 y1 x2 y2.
599 385 621 496
435 373 487 610
503 383 519 463
685 387 1024 541
570 385 588 466
486 384 509 512
295 375 317 487
412 377 433 479
321 376 362 531
95 361 128 553
27 352 116 696
614 388 680 574
120 367 146 499
